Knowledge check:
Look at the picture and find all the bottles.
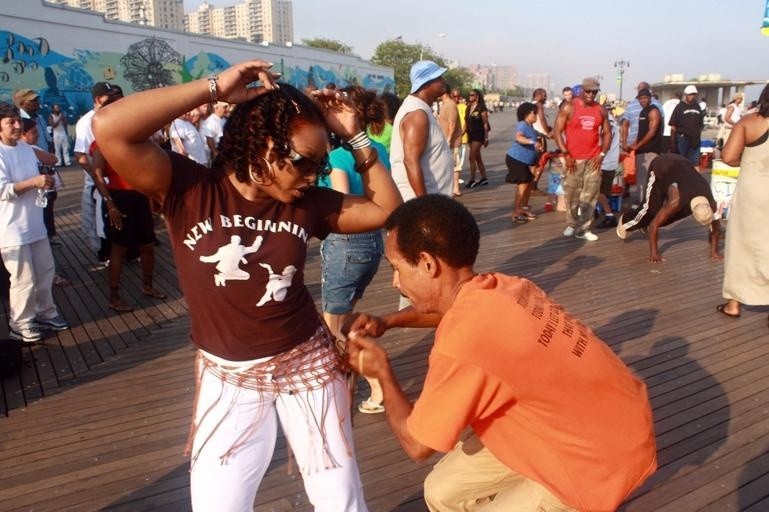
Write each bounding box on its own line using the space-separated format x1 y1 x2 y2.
35 172 48 208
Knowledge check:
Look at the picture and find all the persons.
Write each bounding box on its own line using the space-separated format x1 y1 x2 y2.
305 83 505 195
75 82 236 311
387 57 459 310
89 60 406 512
337 193 658 512
505 77 759 240
615 152 724 262
719 84 769 318
2 88 72 346
318 82 392 415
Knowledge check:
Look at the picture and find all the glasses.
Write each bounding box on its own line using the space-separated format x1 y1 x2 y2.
468 93 477 97
284 145 331 180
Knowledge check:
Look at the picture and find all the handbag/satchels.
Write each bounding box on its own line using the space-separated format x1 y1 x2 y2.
711 159 740 221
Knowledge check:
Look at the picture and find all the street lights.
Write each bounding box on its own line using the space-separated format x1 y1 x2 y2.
614 60 631 99
419 31 447 60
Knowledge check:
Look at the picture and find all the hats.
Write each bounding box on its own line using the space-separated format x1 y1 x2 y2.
409 60 449 94
690 195 714 225
732 93 743 100
13 88 40 105
684 85 698 94
583 78 601 92
92 81 118 99
636 89 650 98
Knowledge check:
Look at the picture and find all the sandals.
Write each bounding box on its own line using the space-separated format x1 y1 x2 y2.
52 273 69 286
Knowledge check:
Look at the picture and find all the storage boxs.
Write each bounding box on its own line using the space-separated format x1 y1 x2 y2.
545 151 628 214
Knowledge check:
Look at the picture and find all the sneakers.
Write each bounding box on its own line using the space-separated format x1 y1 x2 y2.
32 318 70 331
511 187 647 242
465 180 477 187
10 327 41 343
478 178 488 185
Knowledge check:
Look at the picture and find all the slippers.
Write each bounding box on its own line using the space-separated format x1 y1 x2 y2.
108 302 132 313
142 288 165 299
358 397 385 414
716 303 740 318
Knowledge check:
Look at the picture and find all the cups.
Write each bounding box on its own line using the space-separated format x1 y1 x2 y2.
544 202 553 212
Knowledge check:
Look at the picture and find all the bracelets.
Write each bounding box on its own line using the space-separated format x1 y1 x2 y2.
206 74 219 104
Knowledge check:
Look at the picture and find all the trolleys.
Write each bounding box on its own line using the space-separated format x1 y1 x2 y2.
545 150 625 221
